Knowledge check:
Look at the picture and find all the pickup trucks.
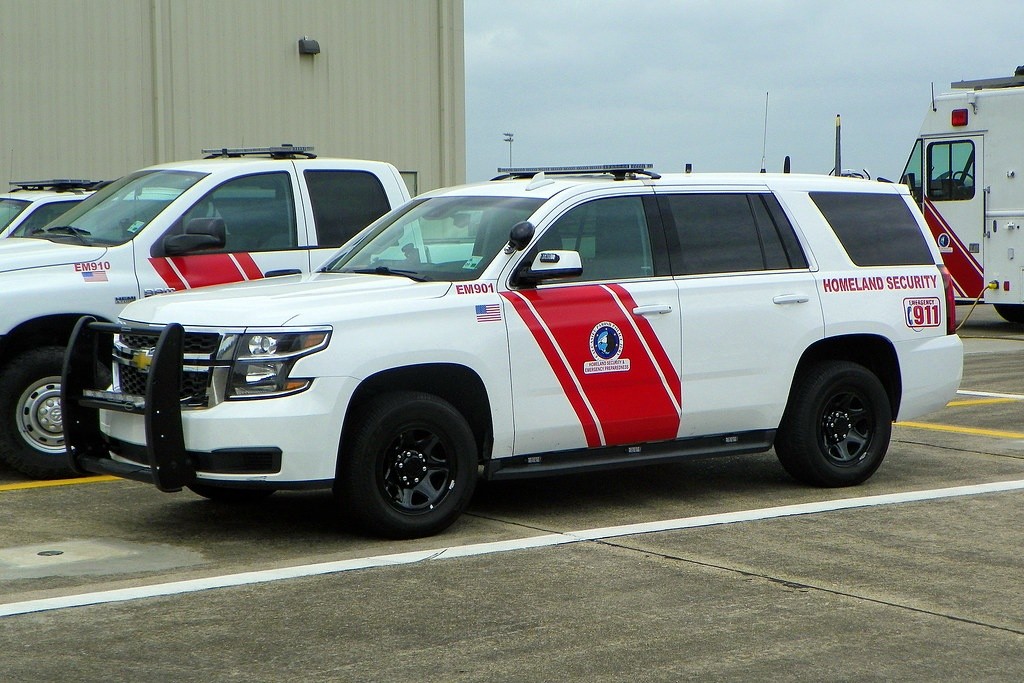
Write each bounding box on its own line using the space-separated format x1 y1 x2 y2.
0 144 596 481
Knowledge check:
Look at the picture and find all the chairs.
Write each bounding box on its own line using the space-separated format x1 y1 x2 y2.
227 199 330 254
470 207 564 274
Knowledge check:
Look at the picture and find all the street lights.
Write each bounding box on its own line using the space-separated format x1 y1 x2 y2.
503 132 513 180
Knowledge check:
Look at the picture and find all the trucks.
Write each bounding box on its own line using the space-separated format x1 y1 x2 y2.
897 65 1024 322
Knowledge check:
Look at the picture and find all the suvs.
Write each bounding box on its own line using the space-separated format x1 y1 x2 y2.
60 156 964 538
